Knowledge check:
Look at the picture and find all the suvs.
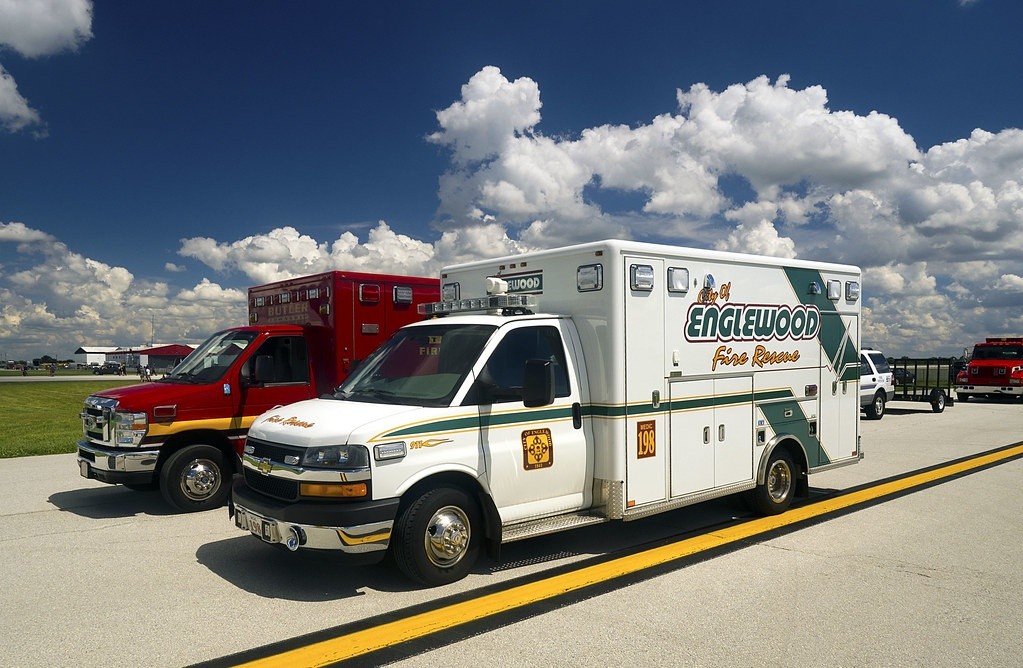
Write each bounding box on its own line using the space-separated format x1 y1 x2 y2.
94 363 121 376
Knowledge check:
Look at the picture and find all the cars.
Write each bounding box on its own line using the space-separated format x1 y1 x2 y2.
890 368 916 386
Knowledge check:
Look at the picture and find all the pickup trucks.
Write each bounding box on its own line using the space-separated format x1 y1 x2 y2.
859 350 895 420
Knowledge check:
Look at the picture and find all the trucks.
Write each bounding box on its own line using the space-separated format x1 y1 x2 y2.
77 270 445 512
953 338 1023 403
229 241 863 589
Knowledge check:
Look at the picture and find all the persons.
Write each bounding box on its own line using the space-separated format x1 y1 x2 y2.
136 361 159 383
